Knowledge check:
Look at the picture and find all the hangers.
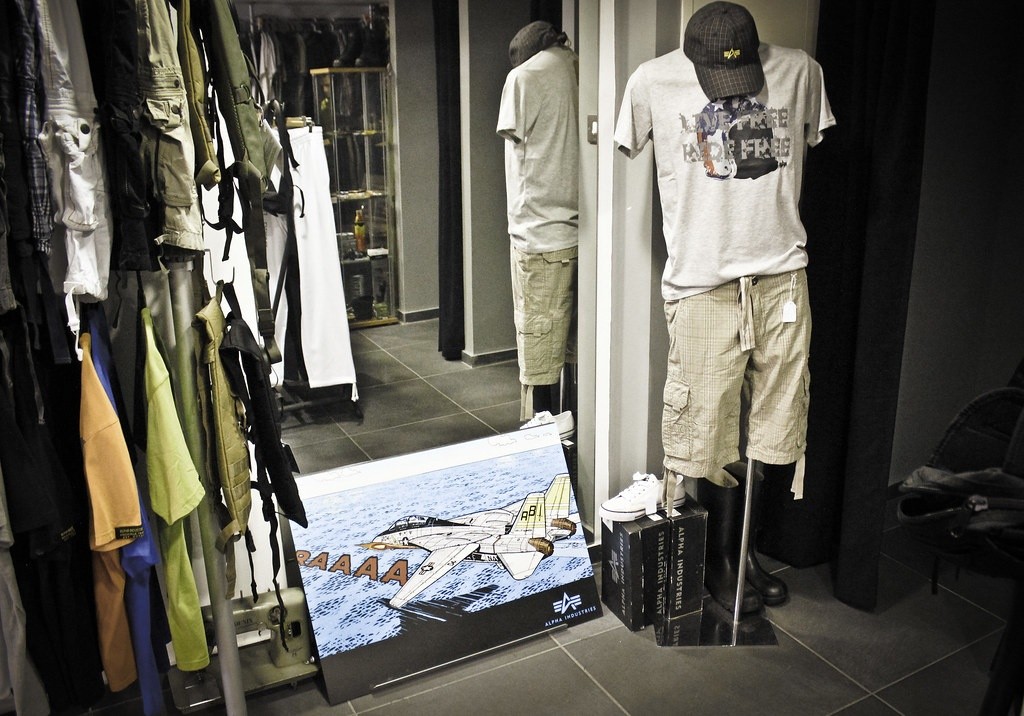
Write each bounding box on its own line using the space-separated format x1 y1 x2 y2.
80 303 91 333
259 15 369 34
135 271 147 328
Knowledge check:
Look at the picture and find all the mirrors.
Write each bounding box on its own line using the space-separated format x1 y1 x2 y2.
247 0 601 590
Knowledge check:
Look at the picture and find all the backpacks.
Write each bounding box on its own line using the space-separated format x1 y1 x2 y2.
896 387 1024 594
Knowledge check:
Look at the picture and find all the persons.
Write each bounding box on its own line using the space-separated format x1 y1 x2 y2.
496 22 579 423
613 0 840 520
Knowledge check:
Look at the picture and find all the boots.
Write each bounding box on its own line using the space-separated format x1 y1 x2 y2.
724 461 788 606
699 468 763 614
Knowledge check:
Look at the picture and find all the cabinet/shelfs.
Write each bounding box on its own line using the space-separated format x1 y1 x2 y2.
308 67 398 330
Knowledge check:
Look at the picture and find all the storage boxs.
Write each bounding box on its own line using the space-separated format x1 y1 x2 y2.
656 491 708 621
600 491 671 632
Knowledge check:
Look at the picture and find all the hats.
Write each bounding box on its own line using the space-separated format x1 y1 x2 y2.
684 1 764 102
509 21 567 69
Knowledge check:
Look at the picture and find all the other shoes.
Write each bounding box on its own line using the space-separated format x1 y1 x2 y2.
520 411 575 441
599 471 686 522
